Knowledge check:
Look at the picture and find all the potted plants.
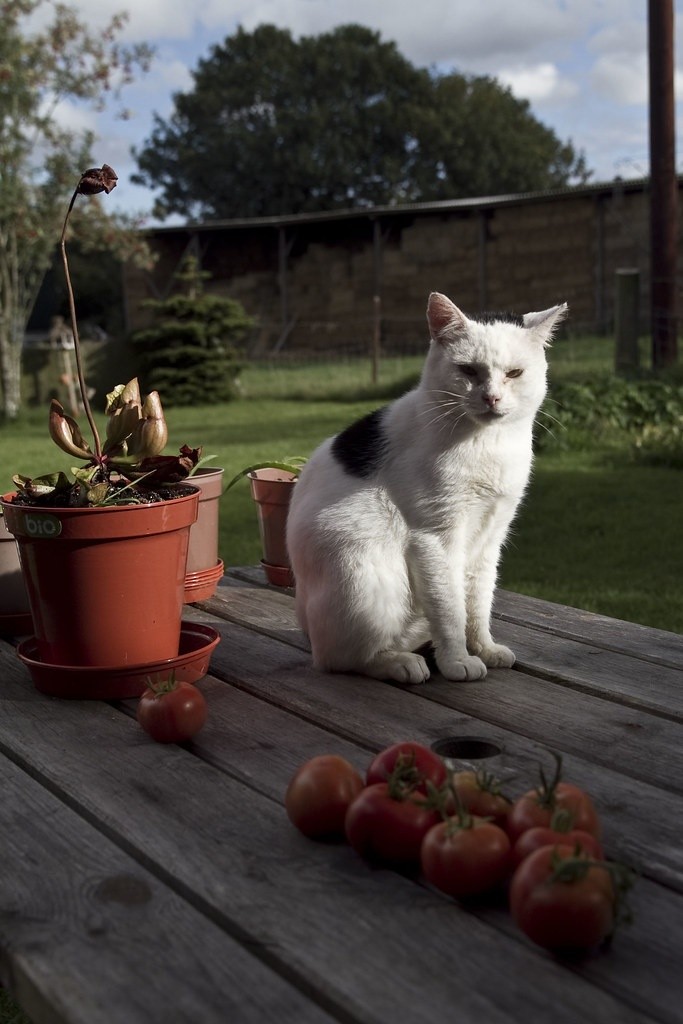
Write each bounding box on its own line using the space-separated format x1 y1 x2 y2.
222 456 309 588
170 456 225 605
0 163 222 700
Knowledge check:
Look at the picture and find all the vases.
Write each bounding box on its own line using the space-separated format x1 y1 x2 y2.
0 501 32 636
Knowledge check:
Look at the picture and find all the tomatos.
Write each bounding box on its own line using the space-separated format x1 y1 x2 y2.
136 670 208 743
285 740 614 954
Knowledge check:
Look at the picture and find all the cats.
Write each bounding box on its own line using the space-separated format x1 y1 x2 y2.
284 291 570 684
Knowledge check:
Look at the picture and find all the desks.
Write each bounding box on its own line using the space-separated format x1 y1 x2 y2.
0 560 683 1024
